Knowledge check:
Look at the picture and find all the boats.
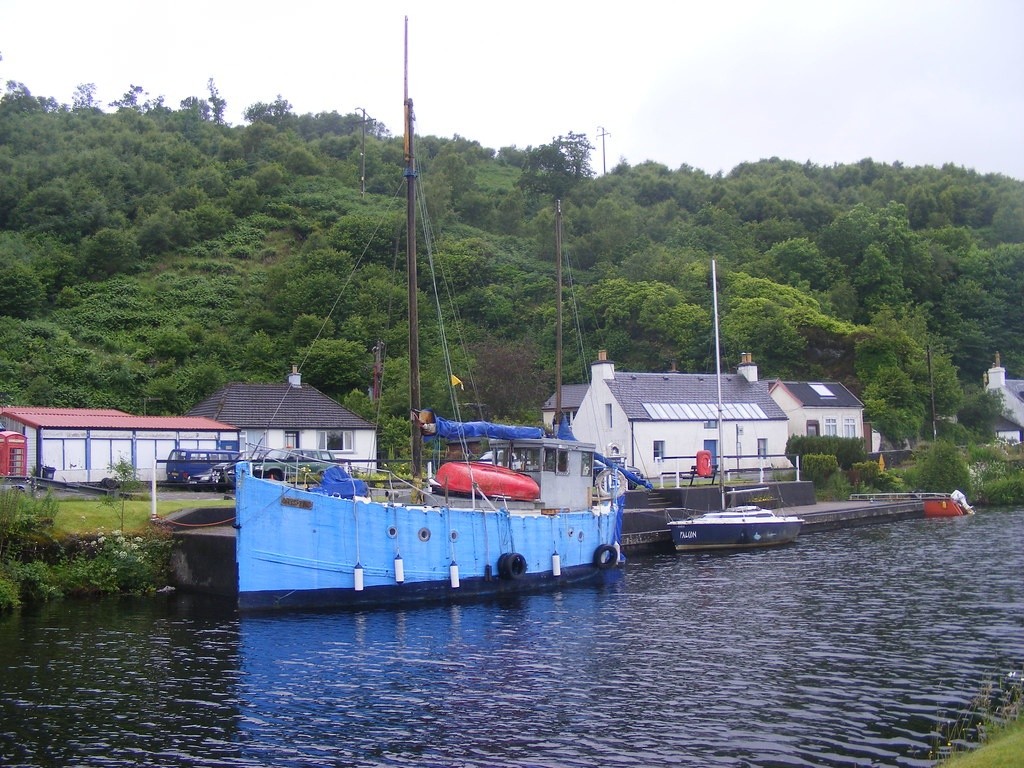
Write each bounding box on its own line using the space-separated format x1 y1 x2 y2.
432 460 540 503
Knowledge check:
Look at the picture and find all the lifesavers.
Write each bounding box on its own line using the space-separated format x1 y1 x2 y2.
593 543 618 571
594 469 627 498
497 552 527 581
252 465 286 482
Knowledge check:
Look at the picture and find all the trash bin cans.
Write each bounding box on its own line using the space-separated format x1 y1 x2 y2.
42 466 56 480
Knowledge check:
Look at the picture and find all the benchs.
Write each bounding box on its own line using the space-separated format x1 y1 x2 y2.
681 465 719 486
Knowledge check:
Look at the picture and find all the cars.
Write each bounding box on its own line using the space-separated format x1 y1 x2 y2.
187 461 229 492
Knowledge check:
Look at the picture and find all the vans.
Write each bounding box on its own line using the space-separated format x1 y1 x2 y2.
165 448 245 485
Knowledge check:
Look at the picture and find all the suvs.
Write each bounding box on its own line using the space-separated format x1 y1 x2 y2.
228 448 338 485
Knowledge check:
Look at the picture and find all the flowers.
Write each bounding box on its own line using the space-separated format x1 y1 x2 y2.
749 493 774 501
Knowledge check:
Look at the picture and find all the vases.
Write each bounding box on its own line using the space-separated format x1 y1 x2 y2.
746 498 777 510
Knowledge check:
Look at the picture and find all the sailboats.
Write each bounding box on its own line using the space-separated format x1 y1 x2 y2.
172 12 632 610
667 260 806 552
845 342 974 517
